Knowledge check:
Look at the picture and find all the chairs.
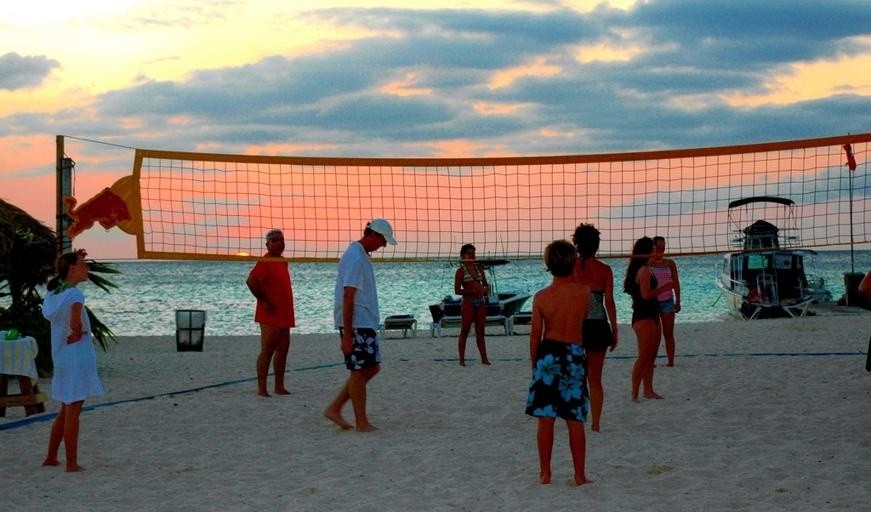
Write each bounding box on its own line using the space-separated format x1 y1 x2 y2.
380 314 419 340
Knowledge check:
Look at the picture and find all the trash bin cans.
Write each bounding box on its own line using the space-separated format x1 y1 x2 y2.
175 310 205 351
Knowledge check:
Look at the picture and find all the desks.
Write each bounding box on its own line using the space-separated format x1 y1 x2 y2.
0 335 50 419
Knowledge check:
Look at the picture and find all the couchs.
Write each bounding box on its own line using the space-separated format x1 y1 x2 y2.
428 291 535 339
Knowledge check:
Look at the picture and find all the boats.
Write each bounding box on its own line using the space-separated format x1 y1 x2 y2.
429 288 531 322
715 195 830 316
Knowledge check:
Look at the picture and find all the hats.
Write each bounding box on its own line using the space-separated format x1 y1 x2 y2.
366 219 398 246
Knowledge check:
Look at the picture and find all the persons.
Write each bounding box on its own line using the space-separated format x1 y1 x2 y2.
247 229 295 398
323 219 399 431
624 236 669 405
527 237 591 490
651 235 680 368
40 247 105 473
858 269 871 372
569 222 621 431
454 244 493 366
